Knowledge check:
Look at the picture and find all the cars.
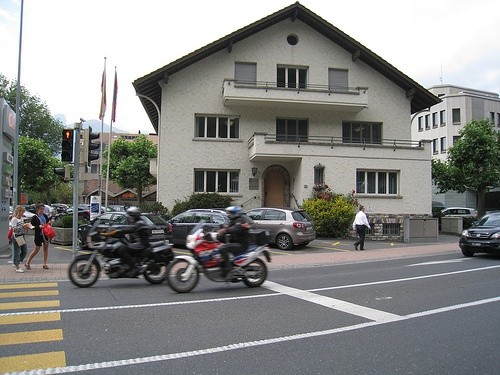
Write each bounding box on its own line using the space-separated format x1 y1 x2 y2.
166 208 231 249
458 209 500 257
441 206 479 222
243 206 316 251
9 203 140 223
86 211 176 254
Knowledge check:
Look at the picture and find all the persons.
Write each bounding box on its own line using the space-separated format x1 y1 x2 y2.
100 206 152 278
217 205 256 280
353 206 372 251
24 203 50 270
11 205 32 273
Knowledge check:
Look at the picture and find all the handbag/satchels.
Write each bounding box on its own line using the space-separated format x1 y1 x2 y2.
42 223 56 241
16 236 26 247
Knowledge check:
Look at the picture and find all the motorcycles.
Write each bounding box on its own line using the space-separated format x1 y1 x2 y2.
164 219 272 294
67 220 175 288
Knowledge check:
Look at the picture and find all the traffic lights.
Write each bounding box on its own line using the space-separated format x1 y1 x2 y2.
83 126 100 167
61 128 76 164
54 164 70 182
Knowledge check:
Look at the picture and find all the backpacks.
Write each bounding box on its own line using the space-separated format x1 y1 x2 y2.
8 227 14 240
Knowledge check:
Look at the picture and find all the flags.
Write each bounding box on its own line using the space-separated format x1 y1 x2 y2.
99 71 107 120
112 71 118 122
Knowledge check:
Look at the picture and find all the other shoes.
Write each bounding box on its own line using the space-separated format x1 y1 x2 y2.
43 264 49 269
24 263 32 270
125 268 139 276
12 264 20 267
224 272 233 280
360 248 365 250
354 243 359 251
16 268 23 273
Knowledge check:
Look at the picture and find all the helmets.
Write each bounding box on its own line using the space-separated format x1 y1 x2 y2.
127 207 141 221
225 206 242 220
